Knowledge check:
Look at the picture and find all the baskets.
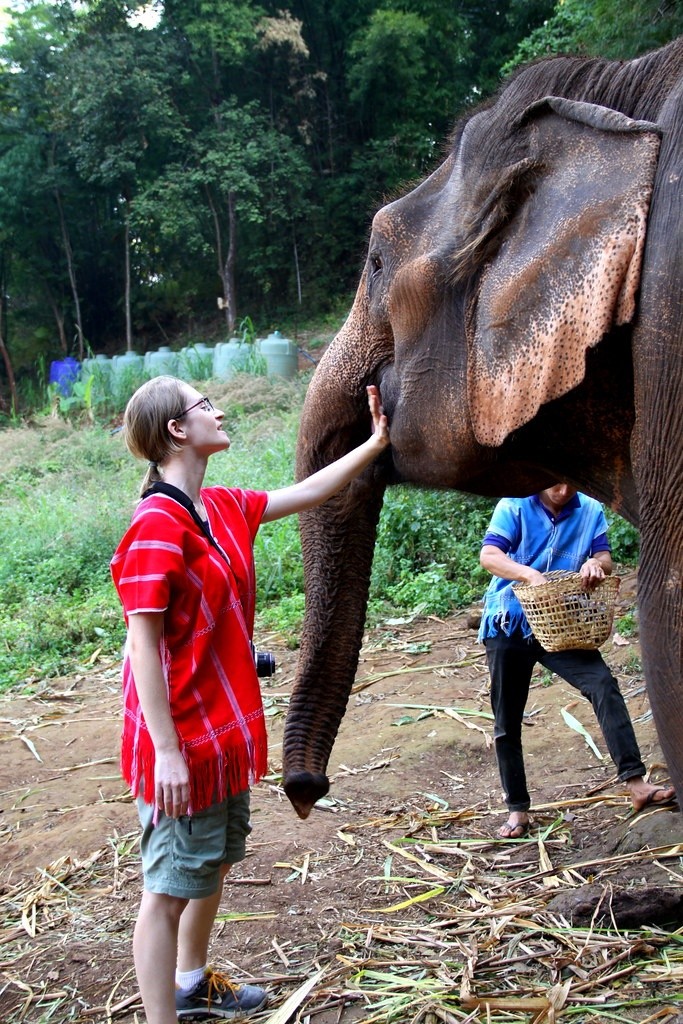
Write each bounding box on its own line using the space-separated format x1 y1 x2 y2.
512 570 622 653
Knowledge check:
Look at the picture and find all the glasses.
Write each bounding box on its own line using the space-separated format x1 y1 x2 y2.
172 396 215 419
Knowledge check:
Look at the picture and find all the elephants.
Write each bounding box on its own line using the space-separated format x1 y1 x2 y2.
278 37 683 820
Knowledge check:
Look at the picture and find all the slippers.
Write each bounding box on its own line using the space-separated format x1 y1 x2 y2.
642 784 678 810
495 819 529 839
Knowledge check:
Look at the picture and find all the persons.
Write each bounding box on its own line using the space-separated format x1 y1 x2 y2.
477 482 677 838
108 375 392 1024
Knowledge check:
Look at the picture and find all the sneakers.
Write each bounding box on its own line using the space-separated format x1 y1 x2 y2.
174 964 268 1019
